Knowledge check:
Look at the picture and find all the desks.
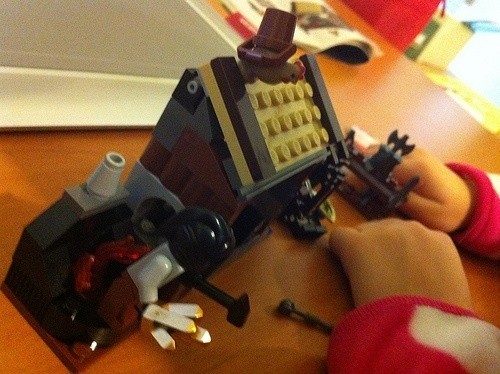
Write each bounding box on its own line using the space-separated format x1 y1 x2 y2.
1 0 499 373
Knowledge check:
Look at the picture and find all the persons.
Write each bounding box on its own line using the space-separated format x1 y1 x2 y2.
327 143 499 374
